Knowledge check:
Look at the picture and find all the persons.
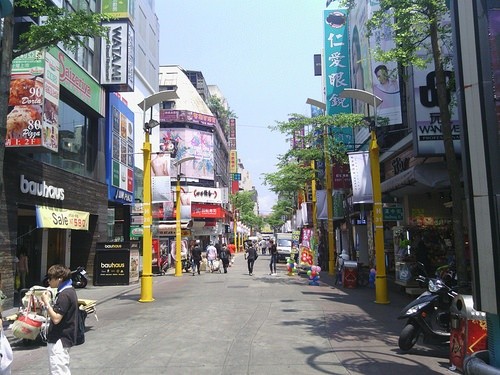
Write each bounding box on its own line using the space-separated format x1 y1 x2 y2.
41 264 79 375
0 312 14 375
338 249 350 285
190 237 279 276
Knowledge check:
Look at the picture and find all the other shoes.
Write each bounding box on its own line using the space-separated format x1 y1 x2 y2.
198 272 200 274
249 273 252 276
224 270 227 273
193 273 195 276
210 269 213 273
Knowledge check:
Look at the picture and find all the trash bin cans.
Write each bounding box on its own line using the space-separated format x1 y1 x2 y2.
448 294 488 373
342 260 358 289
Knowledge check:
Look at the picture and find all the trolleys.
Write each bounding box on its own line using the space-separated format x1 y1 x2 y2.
18 288 97 344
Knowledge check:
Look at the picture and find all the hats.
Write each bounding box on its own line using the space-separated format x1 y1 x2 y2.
210 241 214 244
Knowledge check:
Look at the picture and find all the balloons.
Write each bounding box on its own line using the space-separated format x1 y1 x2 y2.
307 264 322 282
286 258 298 274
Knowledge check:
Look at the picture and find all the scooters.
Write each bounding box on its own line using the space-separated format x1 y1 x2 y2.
399 274 472 352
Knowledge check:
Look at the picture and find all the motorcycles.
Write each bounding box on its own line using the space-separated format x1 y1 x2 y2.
43 261 92 289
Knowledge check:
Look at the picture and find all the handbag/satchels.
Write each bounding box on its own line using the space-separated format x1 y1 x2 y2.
199 257 202 261
255 254 258 260
12 295 42 340
76 310 85 345
276 255 281 261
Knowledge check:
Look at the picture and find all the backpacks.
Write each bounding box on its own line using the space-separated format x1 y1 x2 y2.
220 248 227 259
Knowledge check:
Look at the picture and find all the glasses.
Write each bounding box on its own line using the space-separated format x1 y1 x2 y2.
45 275 58 282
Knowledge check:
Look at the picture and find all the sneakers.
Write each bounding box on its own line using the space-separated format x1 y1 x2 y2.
268 272 273 275
271 273 277 275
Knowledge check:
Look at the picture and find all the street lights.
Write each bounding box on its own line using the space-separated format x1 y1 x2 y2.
176 173 185 275
362 117 389 303
138 119 159 300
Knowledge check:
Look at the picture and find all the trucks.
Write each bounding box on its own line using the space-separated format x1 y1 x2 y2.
276 233 292 262
